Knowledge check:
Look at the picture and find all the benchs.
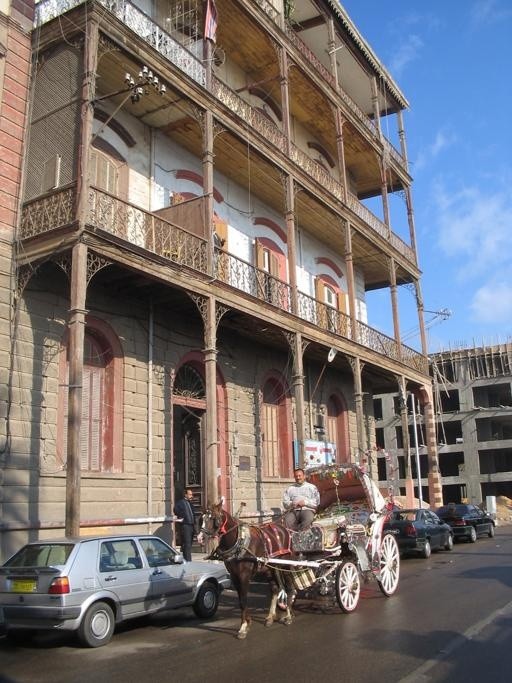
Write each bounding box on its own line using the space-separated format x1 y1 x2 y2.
321 499 372 553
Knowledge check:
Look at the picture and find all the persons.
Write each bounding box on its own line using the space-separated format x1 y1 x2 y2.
172 487 197 561
279 468 321 534
211 220 227 276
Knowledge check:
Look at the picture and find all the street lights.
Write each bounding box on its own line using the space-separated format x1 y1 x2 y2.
296 347 338 469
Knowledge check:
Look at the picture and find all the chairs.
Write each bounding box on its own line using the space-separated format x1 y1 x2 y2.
107 551 136 570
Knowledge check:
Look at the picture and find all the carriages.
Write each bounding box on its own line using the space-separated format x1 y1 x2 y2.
197 463 400 639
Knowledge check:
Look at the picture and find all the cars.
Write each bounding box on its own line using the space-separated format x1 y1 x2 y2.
0 535 231 648
436 504 495 543
382 509 454 558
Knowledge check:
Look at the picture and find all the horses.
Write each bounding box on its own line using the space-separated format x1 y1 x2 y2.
196 498 298 640
